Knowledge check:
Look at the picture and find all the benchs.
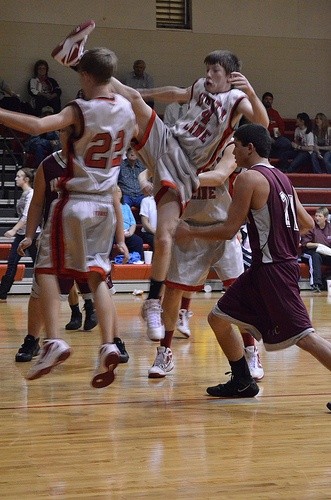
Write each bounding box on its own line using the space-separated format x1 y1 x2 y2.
0 120 331 282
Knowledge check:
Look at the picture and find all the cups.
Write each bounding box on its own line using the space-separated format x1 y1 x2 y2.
273 128 278 137
204 285 212 293
143 251 153 264
327 280 331 292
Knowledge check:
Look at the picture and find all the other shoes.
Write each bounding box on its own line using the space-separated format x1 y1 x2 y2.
133 260 144 264
314 284 321 292
0 291 7 302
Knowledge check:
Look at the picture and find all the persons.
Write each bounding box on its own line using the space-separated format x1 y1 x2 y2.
1 20 331 389
169 123 331 410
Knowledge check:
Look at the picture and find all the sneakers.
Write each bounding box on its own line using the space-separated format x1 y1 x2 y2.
66 313 82 330
27 338 71 380
84 312 97 331
15 334 40 362
140 298 166 341
206 372 259 397
176 307 193 337
51 19 96 67
113 337 129 363
148 346 174 378
91 343 121 388
244 346 264 381
